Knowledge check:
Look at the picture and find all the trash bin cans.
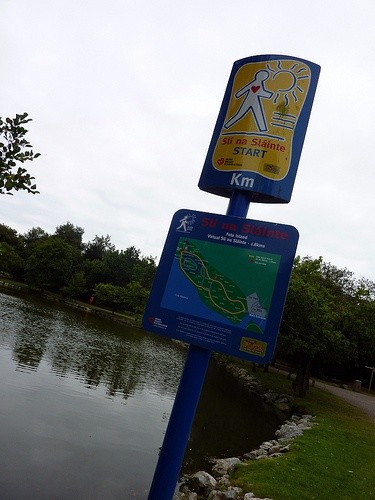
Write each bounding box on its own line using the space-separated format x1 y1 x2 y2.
352 380 362 392
